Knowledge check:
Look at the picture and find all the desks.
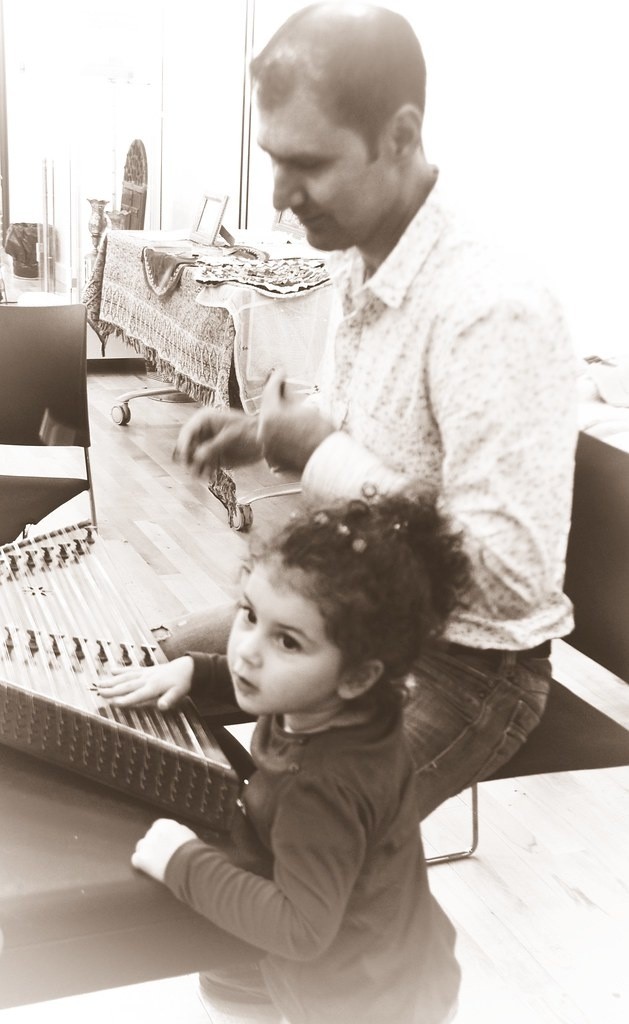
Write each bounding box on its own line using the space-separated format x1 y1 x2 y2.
0 724 274 1013
81 229 334 531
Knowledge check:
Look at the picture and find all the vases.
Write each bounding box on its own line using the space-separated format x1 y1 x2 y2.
86 199 110 257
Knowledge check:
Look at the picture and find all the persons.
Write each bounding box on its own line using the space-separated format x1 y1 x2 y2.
131 478 475 1024
160 4 579 825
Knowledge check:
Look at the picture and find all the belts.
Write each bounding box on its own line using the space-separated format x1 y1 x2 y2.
434 641 552 661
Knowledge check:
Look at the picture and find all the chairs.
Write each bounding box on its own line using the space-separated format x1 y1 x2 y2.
0 303 97 547
422 431 629 867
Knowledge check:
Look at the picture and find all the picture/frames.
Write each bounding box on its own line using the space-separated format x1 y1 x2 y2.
189 193 229 245
274 207 308 238
